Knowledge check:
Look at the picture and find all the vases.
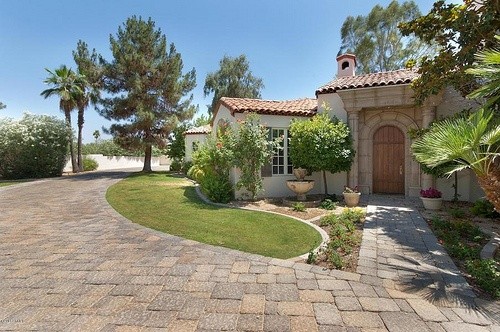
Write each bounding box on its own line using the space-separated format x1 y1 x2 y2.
420 196 443 210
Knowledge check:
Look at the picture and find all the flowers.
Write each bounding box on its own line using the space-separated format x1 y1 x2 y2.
420 187 442 198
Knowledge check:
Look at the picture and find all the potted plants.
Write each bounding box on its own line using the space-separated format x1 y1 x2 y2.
342 185 362 207
